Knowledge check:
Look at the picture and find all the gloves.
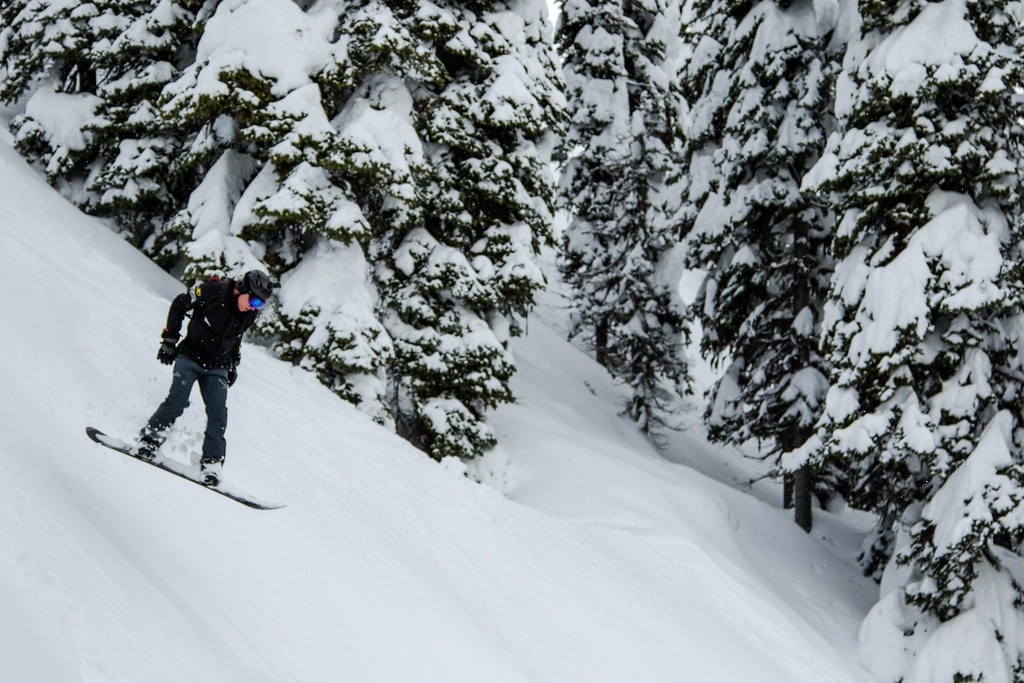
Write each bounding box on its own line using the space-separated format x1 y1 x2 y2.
157 337 178 365
228 361 238 388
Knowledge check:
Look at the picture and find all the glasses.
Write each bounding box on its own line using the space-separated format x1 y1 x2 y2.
248 291 264 309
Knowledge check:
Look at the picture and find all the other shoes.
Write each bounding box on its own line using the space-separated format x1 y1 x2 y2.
200 456 224 486
137 423 165 458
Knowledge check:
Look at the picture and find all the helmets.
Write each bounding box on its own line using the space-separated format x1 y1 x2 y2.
245 270 272 304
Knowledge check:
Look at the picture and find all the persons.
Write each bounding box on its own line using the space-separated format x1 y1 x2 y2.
136 270 274 487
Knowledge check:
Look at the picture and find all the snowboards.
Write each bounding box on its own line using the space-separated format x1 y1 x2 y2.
85 427 288 511
